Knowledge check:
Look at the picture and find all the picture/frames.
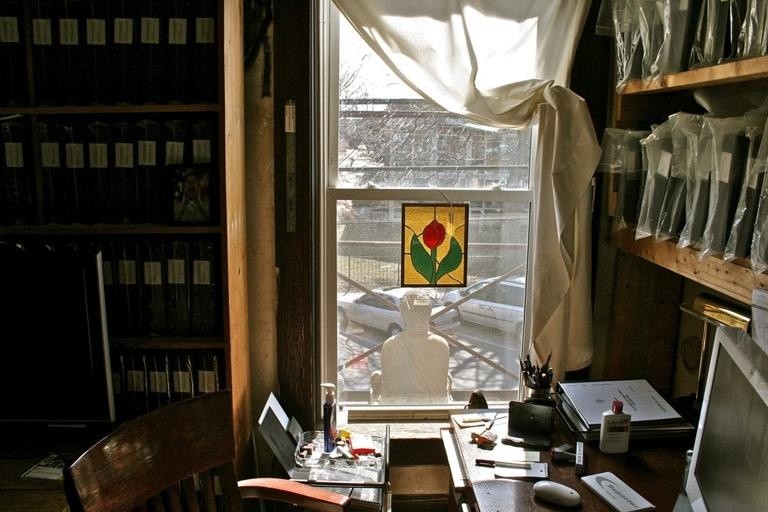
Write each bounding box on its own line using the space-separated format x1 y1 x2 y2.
399 200 472 290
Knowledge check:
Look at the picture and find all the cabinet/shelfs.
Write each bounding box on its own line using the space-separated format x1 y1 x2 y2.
593 0 767 512
0 0 324 511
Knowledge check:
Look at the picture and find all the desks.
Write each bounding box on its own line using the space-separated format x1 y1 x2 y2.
441 415 694 512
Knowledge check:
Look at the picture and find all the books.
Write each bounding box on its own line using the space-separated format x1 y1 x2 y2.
554 377 698 449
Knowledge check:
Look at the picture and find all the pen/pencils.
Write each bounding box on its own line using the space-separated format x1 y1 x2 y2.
519 349 553 388
476 459 531 469
463 417 490 422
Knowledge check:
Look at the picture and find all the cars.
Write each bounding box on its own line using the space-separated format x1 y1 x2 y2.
337 276 525 345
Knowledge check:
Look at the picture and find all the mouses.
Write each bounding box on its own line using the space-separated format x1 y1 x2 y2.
533 480 582 506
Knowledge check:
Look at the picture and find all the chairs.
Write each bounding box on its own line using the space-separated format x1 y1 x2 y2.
61 398 352 512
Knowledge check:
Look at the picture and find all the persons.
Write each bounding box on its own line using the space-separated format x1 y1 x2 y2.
368 291 456 406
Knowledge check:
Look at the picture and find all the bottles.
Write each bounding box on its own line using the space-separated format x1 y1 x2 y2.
600 401 631 454
336 404 348 431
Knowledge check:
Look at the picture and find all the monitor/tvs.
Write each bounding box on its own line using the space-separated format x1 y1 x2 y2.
685 325 768 512
